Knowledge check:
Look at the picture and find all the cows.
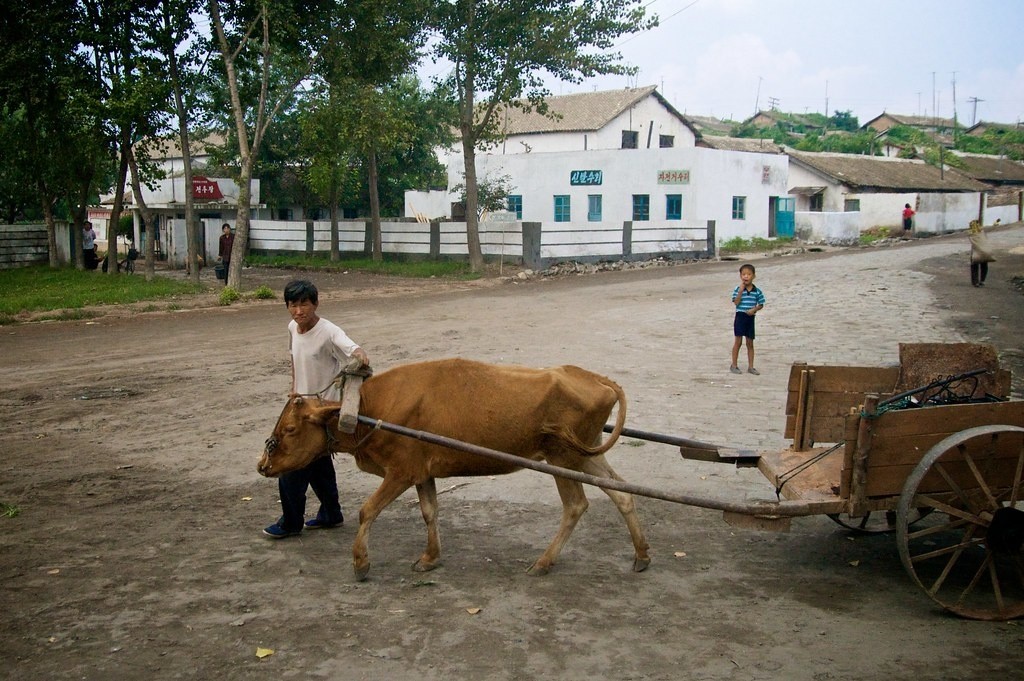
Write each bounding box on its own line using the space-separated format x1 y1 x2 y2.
256 357 651 582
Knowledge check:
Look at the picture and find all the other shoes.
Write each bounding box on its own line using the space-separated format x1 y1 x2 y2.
975 283 979 287
730 365 741 373
980 282 985 286
748 367 759 375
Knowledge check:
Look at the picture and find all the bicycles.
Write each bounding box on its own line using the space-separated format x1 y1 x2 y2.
117 243 144 276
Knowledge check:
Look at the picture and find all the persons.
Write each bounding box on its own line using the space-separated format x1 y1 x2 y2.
102 255 122 273
217 223 234 285
82 221 96 273
903 203 915 234
94 244 105 270
730 264 765 375
970 220 988 288
262 279 370 538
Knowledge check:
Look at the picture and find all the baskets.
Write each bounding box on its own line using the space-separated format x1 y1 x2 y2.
128 250 139 260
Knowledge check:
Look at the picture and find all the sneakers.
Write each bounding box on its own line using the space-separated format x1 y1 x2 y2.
263 523 302 537
305 518 344 529
